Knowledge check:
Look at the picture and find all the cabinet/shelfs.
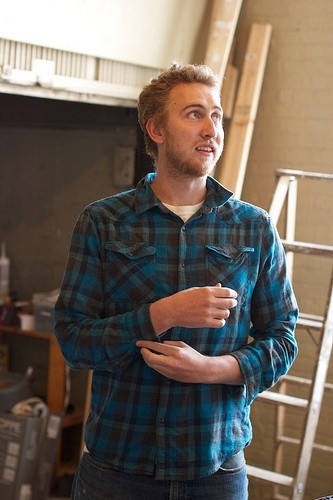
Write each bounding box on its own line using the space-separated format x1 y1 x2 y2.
0 323 92 491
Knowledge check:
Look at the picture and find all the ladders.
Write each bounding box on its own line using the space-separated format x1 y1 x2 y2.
245 168 333 500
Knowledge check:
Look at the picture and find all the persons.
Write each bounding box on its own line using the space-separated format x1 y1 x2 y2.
53 62 299 500
